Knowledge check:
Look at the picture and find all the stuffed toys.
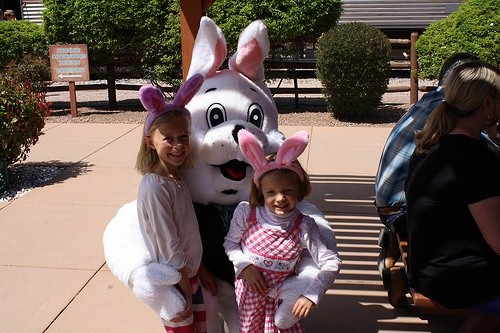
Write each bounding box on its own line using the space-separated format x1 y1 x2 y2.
103 13 341 333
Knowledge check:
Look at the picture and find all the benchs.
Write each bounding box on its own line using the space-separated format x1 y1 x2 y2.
389 223 500 333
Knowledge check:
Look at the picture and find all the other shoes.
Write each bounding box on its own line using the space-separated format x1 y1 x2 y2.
377 227 400 278
381 267 410 308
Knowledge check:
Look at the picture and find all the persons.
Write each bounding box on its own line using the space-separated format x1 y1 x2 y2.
133 73 207 333
221 128 341 333
0 9 17 22
373 53 500 320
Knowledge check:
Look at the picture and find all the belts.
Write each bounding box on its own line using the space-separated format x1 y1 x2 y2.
377 206 403 215
